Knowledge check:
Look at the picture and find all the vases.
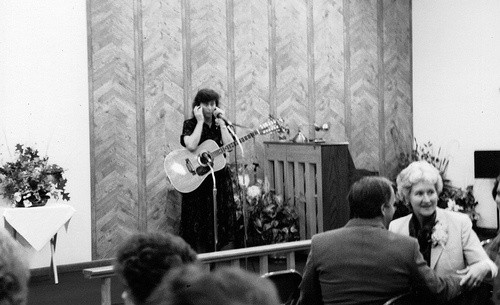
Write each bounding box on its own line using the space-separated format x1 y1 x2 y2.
20 195 50 208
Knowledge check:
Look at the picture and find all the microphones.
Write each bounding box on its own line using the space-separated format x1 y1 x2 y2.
214 109 233 126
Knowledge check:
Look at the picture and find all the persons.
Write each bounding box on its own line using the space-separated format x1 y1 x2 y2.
120 228 281 305
0 227 31 305
296 158 500 305
179 88 238 254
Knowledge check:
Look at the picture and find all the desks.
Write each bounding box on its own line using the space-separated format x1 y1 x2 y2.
3 206 72 285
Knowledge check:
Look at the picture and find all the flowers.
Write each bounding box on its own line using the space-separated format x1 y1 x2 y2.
228 163 300 241
444 184 481 222
2 144 72 208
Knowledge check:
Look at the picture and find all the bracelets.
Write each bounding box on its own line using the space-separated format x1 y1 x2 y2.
220 125 226 129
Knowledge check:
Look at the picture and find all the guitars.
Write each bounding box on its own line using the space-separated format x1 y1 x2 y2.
163 116 287 194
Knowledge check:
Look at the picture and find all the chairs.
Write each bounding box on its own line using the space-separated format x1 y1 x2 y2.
260 269 302 305
